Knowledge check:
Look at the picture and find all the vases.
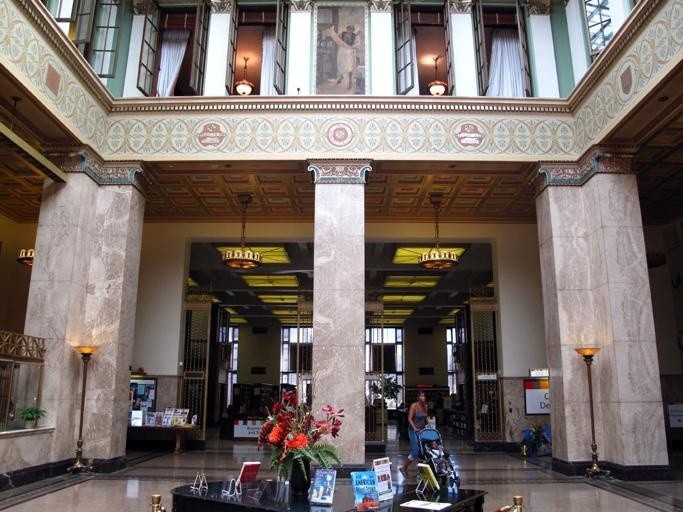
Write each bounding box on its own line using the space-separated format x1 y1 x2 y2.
289 452 312 493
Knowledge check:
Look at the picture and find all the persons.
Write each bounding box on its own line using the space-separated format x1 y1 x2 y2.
398 389 433 479
335 25 357 91
429 438 454 477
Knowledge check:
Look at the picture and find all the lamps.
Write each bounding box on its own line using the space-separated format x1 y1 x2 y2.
233 55 255 96
218 193 263 272
417 193 459 272
14 246 36 268
427 53 448 96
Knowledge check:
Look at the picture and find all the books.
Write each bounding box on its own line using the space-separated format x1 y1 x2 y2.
400 499 452 511
308 467 337 507
372 457 394 502
350 469 381 512
128 398 190 427
235 461 262 492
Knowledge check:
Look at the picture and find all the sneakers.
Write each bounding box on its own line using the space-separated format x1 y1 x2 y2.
399 467 408 479
442 470 454 475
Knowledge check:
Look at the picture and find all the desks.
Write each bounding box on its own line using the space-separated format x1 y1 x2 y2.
170 479 489 512
127 425 193 455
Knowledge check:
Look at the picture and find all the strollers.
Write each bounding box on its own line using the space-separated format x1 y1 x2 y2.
416 428 460 488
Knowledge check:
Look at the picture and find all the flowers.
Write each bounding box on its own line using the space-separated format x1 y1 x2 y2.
255 387 346 475
